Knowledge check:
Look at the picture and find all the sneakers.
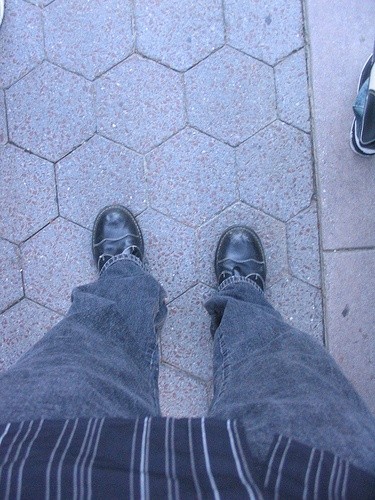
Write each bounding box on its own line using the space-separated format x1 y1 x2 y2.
350 53 375 157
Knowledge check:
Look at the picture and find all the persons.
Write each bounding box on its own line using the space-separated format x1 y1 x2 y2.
0 203 374 500
348 41 375 158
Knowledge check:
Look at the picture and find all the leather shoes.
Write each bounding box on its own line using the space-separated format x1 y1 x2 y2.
214 224 266 292
91 204 144 274
